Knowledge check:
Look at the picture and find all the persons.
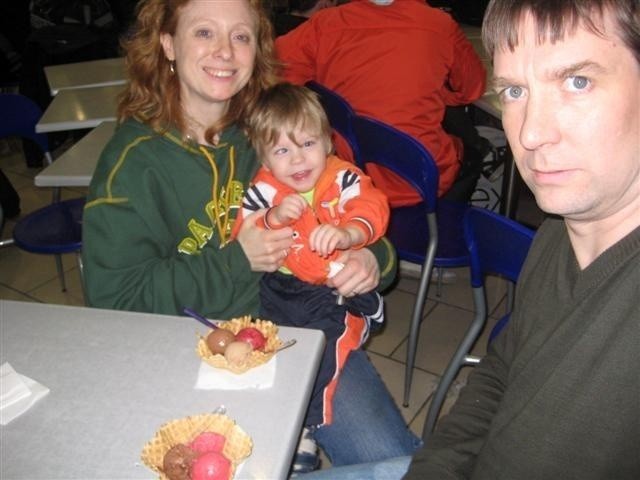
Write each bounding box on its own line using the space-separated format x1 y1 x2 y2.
268 0 489 256
292 0 640 480
79 0 423 467
228 84 391 472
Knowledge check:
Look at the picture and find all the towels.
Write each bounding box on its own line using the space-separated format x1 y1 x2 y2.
195 354 277 391
0 362 49 425
134 460 246 480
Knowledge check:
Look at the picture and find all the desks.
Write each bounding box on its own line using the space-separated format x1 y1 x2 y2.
33 121 116 187
43 58 126 96
35 86 123 134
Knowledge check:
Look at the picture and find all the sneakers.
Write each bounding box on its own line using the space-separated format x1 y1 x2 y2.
290 421 323 474
395 257 457 284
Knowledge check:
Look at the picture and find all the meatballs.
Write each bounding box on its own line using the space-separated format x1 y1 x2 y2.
156 432 233 480
207 327 268 364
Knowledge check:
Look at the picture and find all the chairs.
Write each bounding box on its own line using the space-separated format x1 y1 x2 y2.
0 93 92 306
